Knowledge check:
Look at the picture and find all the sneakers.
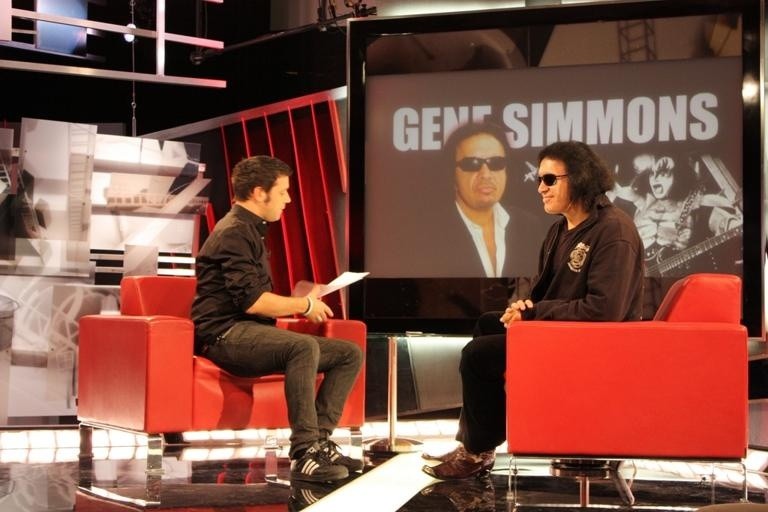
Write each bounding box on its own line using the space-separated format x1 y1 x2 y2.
290 459 349 481
289 482 335 505
319 441 363 472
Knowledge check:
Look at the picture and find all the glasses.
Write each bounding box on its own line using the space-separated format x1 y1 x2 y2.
537 173 569 186
457 156 507 171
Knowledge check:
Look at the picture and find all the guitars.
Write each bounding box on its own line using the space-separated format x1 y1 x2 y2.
642 225 743 284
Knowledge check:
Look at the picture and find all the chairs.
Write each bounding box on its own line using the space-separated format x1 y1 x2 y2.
77 278 367 470
505 274 748 509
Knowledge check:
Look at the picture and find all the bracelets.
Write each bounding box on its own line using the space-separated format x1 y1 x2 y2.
304 297 312 317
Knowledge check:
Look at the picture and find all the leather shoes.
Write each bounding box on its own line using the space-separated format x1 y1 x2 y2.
422 449 495 480
422 481 494 511
421 445 462 462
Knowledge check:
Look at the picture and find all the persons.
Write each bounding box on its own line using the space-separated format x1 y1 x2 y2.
422 140 645 479
413 120 541 278
189 156 366 482
606 151 739 276
